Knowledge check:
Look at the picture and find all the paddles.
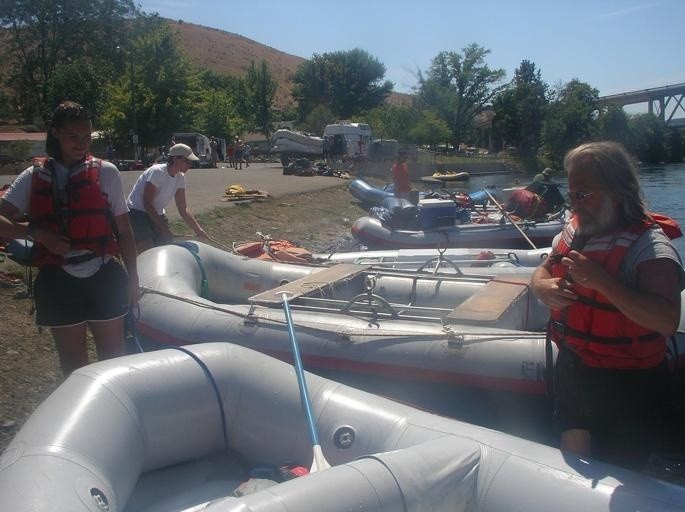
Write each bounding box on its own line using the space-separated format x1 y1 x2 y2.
280 292 331 476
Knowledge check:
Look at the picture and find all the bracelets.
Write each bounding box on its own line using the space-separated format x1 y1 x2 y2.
26 226 35 243
196 228 204 237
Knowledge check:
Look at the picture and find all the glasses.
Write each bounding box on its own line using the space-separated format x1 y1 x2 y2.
61 106 89 117
565 190 593 200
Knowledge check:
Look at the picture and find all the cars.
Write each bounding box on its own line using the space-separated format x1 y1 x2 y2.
107 151 169 171
507 147 520 156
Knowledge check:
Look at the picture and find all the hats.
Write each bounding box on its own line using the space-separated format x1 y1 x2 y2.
542 168 552 176
168 143 200 161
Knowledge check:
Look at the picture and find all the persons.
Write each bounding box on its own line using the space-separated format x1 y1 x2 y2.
502 182 549 223
126 142 211 254
210 134 219 168
319 134 346 169
0 99 142 381
233 135 244 170
227 143 236 167
524 167 565 211
390 147 413 199
527 135 685 462
243 139 252 168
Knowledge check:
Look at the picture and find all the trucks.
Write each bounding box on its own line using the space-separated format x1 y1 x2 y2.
173 132 218 169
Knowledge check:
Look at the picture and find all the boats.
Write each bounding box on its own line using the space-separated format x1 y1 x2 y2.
268 128 323 167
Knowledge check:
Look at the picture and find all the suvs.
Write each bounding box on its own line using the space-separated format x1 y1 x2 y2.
421 144 481 159
